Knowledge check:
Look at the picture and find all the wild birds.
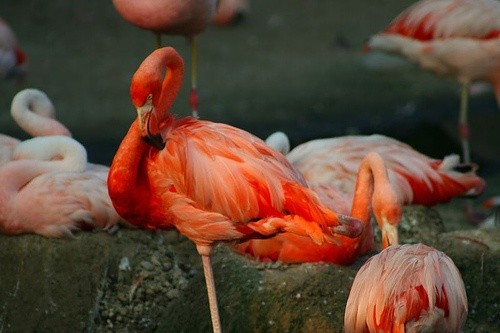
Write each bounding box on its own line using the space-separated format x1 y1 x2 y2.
0 0 500 331
